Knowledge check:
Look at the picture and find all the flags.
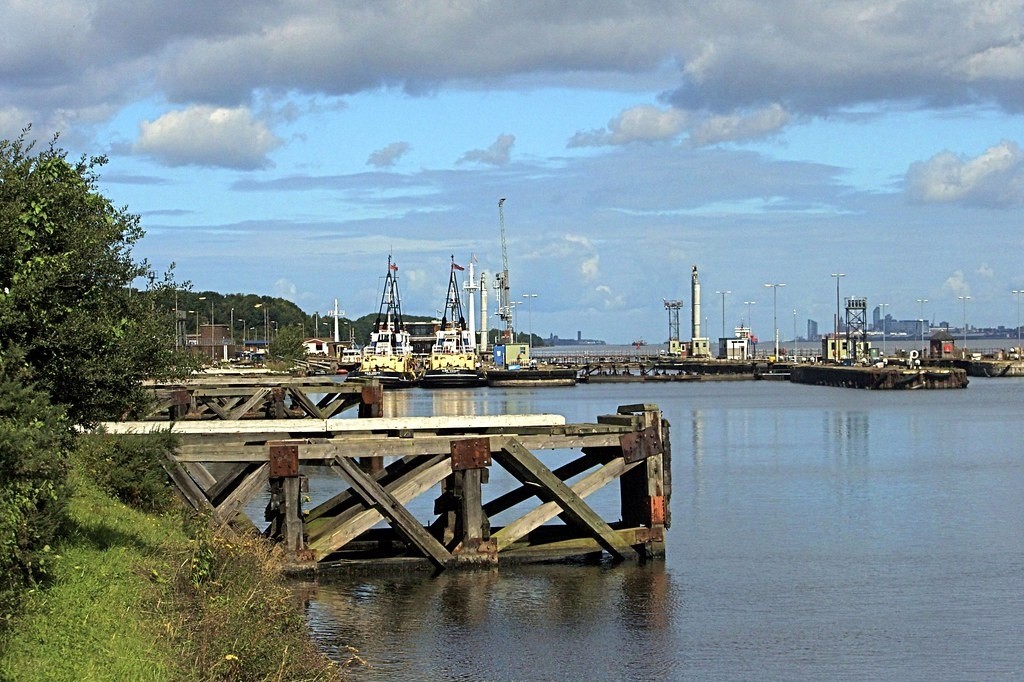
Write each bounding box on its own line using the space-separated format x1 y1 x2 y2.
453 262 464 270
473 255 478 263
390 265 398 270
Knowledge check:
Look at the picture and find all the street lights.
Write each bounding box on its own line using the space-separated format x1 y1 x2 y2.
522 294 538 352
237 318 246 349
199 296 215 362
188 311 198 346
297 323 305 339
878 303 890 354
250 327 258 341
831 273 847 360
253 303 267 347
510 301 524 343
1012 289 1024 348
323 322 332 338
344 321 352 343
745 301 757 361
765 283 786 362
916 300 930 358
715 290 733 339
957 296 972 348
271 321 278 338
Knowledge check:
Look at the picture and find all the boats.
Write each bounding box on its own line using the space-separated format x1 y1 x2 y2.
420 254 487 387
343 244 420 385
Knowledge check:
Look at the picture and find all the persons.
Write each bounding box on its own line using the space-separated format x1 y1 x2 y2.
862 357 866 367
915 358 920 369
375 365 379 372
883 357 888 368
810 355 815 365
475 361 480 370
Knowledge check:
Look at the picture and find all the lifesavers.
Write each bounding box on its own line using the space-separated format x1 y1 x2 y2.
910 350 919 359
